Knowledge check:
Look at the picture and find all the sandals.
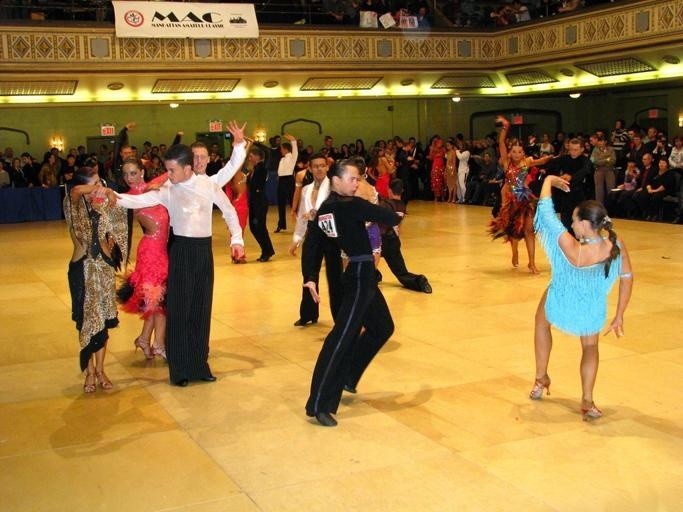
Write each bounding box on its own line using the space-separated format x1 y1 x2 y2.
84 367 113 393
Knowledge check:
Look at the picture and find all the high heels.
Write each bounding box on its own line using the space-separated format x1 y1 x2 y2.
294 319 317 326
344 384 356 393
530 374 550 399
512 261 519 268
231 254 246 264
135 335 167 360
528 263 540 274
306 411 337 426
581 399 603 421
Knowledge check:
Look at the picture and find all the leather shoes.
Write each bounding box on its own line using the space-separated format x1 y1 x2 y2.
257 250 275 262
418 275 432 293
275 226 286 233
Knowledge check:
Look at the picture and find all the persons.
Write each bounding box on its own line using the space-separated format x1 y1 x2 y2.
327 1 579 26
